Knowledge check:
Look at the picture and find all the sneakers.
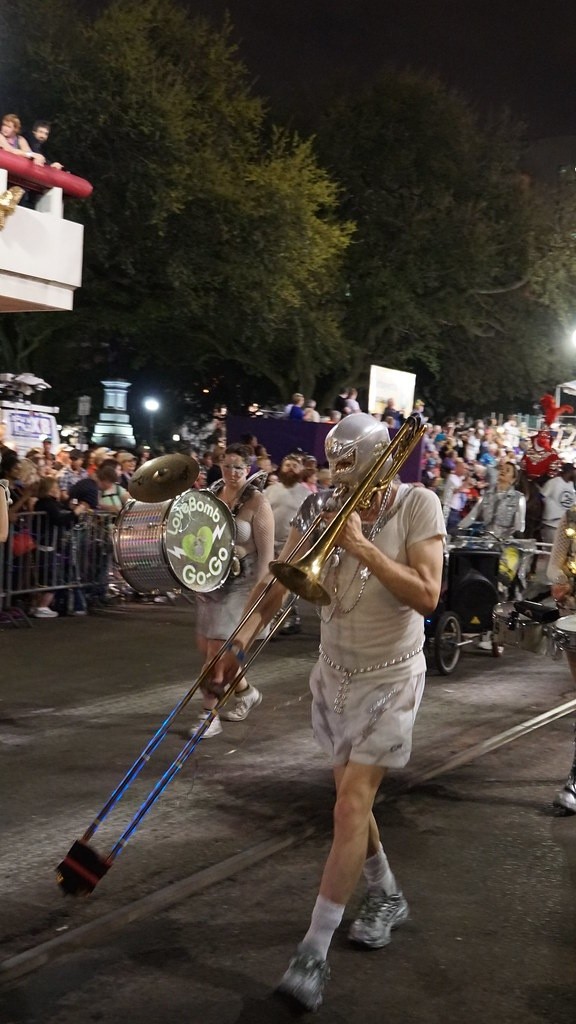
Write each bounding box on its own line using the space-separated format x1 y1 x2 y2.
220 683 263 721
187 710 223 738
347 886 410 948
29 607 58 617
271 943 331 1013
278 615 302 634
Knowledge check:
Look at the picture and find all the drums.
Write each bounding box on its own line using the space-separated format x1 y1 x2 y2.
543 613 576 687
492 599 546 655
110 487 238 597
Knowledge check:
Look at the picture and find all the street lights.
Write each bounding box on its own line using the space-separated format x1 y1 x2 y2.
145 398 159 443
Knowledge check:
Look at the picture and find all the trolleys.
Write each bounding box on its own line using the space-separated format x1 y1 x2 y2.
424 532 506 676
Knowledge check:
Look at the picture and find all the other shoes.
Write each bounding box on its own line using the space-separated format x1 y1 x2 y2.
476 637 494 650
553 773 576 811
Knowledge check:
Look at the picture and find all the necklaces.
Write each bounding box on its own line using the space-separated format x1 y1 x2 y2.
226 490 235 504
315 483 393 624
333 481 392 613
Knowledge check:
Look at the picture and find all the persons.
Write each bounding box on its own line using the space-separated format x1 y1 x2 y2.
0 114 45 167
0 386 576 653
9 120 72 208
190 445 275 739
547 501 576 812
201 412 450 1011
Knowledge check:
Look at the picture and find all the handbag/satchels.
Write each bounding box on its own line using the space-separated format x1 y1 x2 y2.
12 533 37 557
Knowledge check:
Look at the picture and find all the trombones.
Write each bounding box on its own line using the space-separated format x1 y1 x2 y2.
54 412 429 899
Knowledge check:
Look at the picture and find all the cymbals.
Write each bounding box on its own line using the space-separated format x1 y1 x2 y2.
127 453 200 504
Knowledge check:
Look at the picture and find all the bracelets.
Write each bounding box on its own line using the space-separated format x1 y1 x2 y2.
25 151 32 158
227 645 245 661
